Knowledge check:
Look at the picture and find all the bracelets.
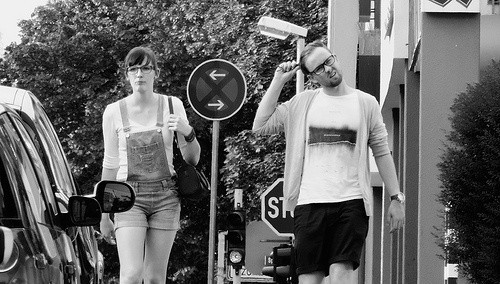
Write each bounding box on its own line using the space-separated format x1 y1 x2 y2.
185 128 196 143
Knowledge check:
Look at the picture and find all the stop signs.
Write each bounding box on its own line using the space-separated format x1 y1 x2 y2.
261 177 294 237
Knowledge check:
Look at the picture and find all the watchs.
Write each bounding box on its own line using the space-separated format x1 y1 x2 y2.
390 192 405 203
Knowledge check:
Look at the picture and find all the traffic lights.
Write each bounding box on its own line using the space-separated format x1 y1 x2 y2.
226 210 246 265
262 244 297 284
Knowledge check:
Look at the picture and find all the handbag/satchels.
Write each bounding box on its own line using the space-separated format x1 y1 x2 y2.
168 97 211 200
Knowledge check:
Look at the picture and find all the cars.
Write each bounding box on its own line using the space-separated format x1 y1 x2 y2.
0 84 137 284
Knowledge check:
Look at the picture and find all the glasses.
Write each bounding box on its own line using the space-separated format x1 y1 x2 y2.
310 55 335 76
126 66 155 74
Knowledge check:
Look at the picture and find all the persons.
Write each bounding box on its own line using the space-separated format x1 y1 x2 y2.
100 47 200 284
251 40 406 284
